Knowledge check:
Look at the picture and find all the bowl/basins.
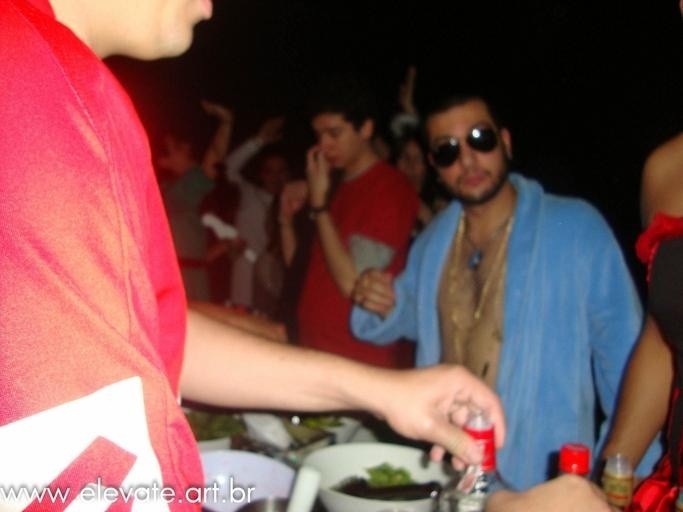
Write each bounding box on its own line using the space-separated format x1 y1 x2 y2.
303 442 457 511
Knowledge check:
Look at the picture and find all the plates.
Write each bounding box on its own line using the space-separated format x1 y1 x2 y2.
199 448 299 511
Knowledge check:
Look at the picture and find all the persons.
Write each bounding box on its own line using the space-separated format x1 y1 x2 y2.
349 82 661 507
126 45 683 368
0 0 612 512
592 136 683 512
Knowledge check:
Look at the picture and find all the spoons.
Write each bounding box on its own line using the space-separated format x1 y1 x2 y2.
337 477 440 500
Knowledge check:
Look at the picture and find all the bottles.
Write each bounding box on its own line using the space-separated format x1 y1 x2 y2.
438 407 523 512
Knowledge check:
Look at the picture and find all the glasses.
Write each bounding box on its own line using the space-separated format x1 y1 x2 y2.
431 121 498 168
